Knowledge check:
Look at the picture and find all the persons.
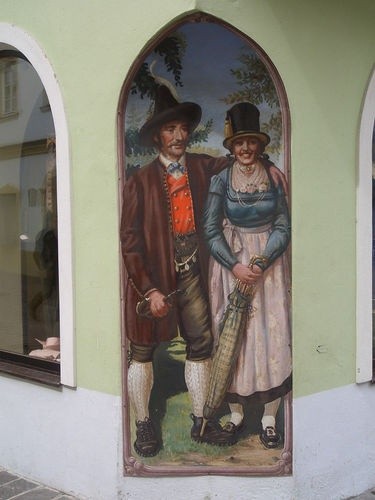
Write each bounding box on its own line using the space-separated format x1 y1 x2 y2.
29 209 59 325
